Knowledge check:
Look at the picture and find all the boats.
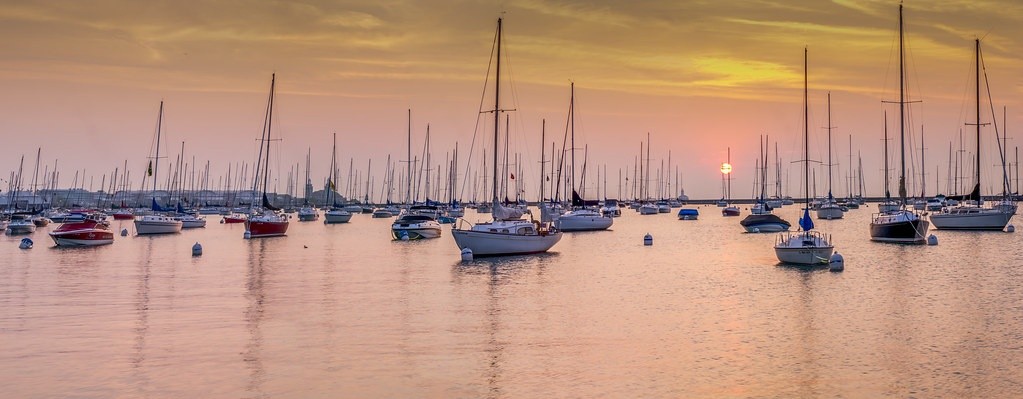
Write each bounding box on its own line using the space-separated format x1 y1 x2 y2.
677 207 700 222
49 219 115 247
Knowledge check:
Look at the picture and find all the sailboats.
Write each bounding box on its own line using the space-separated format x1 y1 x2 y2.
0 74 690 235
714 90 1023 234
772 43 835 266
866 0 930 245
927 38 1019 232
448 13 563 258
